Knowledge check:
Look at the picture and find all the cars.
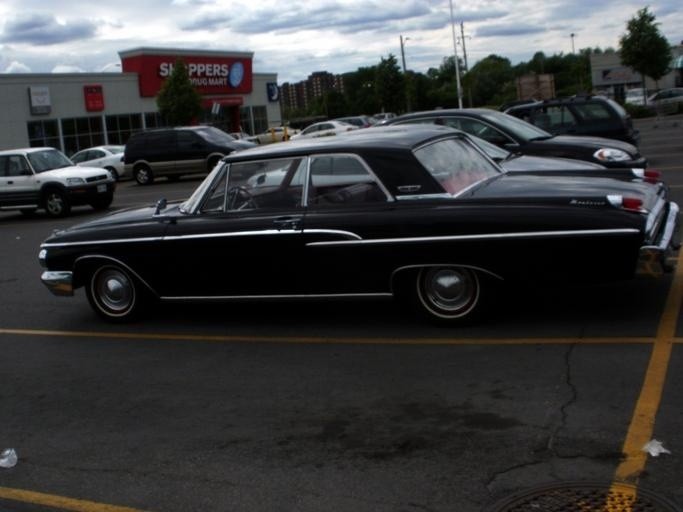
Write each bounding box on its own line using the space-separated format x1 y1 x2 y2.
233 103 660 194
69 143 127 181
35 125 680 330
649 88 680 111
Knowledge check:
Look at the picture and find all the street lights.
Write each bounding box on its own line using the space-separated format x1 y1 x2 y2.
399 34 415 113
455 22 477 109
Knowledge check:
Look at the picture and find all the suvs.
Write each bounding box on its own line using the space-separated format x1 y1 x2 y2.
0 145 117 220
477 93 641 153
120 124 262 187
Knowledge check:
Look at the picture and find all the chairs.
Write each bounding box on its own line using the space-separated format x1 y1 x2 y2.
271 181 373 209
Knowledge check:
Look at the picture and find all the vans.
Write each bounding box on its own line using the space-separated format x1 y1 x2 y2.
625 88 648 110
